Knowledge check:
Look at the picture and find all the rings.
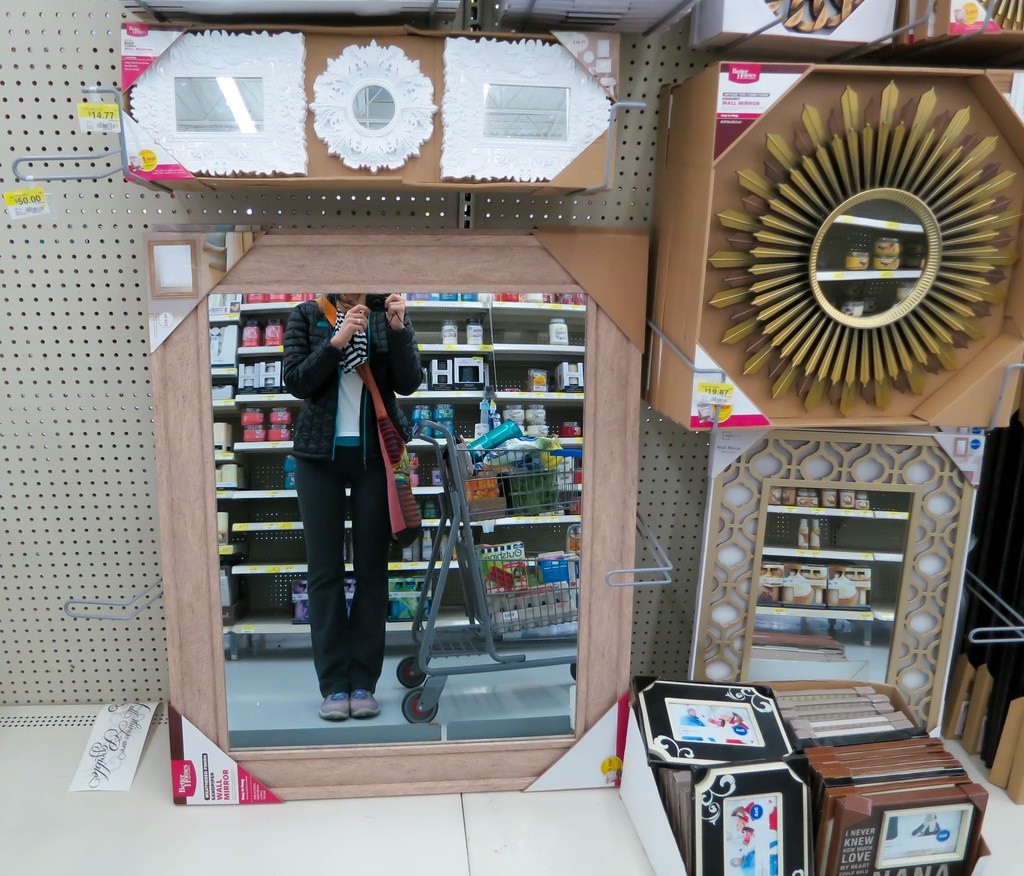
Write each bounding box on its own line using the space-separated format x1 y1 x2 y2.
357 318 362 325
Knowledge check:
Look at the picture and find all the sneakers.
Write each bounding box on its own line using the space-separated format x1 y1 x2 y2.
319 691 350 720
350 689 380 718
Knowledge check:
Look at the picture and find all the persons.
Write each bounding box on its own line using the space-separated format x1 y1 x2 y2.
284 293 425 722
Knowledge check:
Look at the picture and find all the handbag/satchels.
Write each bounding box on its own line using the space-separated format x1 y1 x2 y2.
376 415 421 549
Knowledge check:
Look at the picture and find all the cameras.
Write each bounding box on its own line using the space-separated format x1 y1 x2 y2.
365 294 389 313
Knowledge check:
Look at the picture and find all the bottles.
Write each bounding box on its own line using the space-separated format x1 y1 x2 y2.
811 519 821 548
797 517 808 549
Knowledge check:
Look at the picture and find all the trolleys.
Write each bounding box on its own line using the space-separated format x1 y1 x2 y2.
394 419 584 725
